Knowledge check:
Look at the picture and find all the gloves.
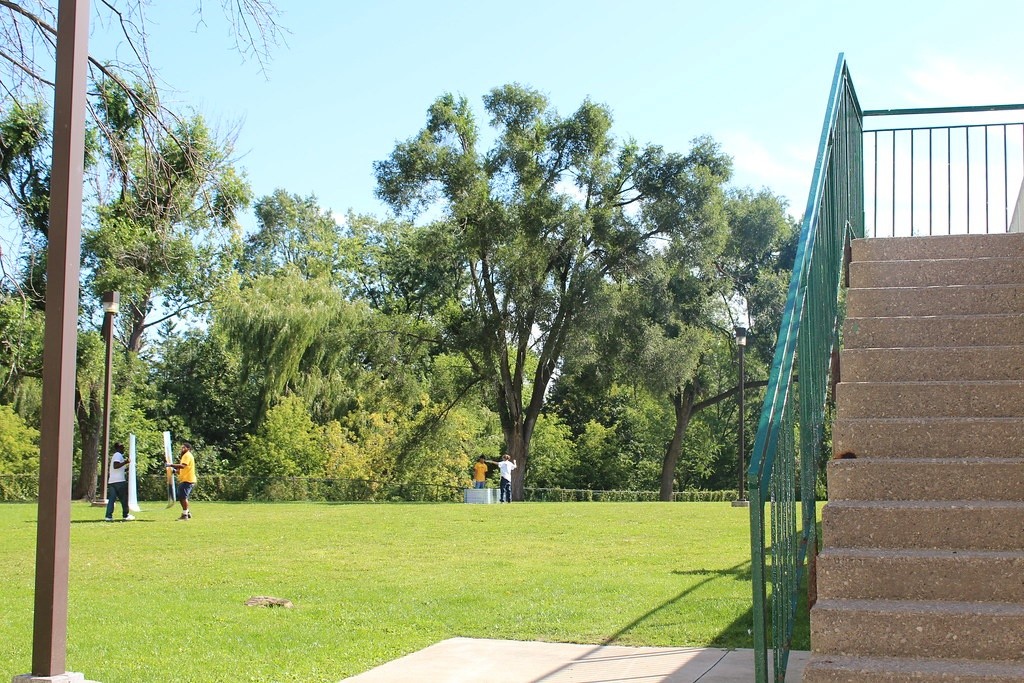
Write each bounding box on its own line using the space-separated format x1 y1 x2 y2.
173 470 176 473
166 463 171 467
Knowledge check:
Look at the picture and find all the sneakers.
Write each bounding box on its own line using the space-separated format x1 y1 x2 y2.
174 513 188 520
105 516 112 520
123 515 135 520
189 512 191 517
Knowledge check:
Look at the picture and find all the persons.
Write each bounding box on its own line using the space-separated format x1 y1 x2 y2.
473 454 488 489
486 454 517 504
105 442 135 521
165 443 196 521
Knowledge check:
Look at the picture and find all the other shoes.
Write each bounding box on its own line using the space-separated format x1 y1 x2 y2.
500 499 503 502
507 500 510 503
